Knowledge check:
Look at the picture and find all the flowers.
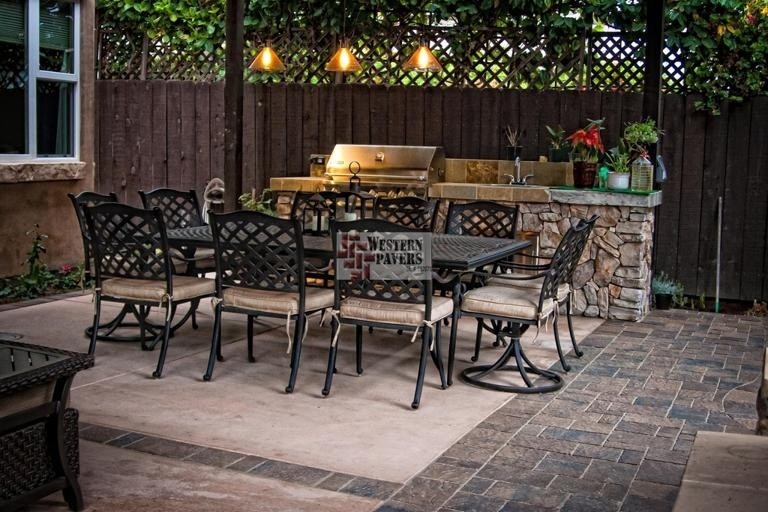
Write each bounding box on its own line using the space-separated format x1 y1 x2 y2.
544 124 602 166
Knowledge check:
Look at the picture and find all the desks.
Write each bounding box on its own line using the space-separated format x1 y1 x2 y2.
134 227 532 386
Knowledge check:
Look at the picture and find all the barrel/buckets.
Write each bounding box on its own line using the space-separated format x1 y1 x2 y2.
631 151 654 193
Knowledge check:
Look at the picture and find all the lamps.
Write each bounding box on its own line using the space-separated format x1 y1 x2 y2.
396 1 443 74
242 1 286 74
322 1 363 74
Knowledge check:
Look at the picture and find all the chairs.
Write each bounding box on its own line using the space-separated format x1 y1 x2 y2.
321 217 448 407
68 175 521 326
458 225 590 393
202 211 347 395
82 201 224 379
487 215 599 373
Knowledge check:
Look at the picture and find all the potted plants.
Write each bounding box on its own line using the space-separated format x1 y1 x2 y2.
605 111 667 195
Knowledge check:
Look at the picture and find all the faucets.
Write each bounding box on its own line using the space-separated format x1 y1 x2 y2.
515 156 522 183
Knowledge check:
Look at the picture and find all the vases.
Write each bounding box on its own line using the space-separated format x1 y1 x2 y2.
567 158 601 188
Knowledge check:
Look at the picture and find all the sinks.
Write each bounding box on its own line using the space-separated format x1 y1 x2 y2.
481 184 531 187
